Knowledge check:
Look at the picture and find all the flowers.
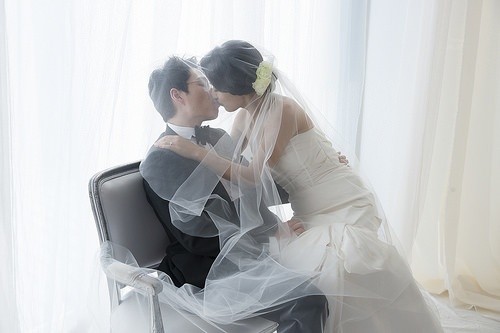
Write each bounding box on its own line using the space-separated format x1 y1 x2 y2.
252 61 273 94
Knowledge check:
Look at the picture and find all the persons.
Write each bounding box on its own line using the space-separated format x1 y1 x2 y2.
140 57 350 333
154 40 444 333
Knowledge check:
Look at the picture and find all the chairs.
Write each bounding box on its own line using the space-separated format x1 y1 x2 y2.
88 161 278 333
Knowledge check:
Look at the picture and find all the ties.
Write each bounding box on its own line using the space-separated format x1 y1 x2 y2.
200 125 210 145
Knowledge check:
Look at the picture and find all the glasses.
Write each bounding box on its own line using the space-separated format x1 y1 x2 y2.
177 78 209 91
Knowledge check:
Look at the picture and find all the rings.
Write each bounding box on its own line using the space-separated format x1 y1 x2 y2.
170 142 173 145
